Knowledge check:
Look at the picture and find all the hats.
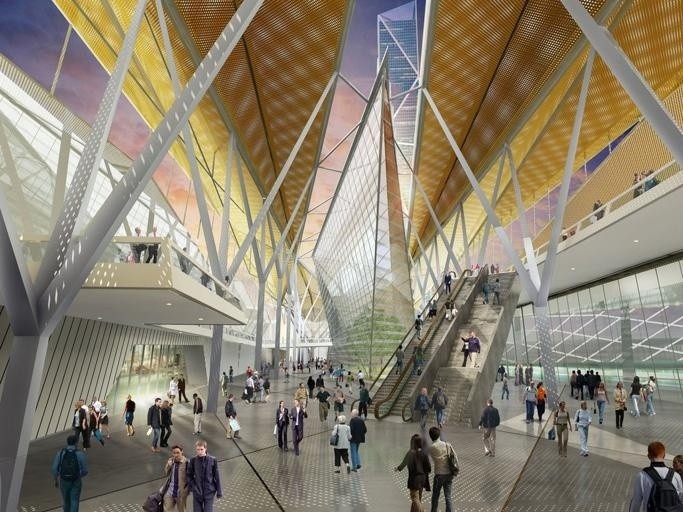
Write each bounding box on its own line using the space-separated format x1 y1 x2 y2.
336 415 346 424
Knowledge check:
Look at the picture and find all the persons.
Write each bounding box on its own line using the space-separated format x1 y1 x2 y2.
633 171 659 199
395 434 431 512
630 443 683 512
673 455 683 478
593 200 605 219
120 395 135 436
426 427 459 512
52 436 88 512
562 227 569 239
71 397 111 450
131 227 146 263
497 363 659 457
181 247 188 270
495 264 499 274
147 357 372 512
491 279 501 305
395 263 483 434
146 226 159 263
490 264 495 275
478 399 499 457
483 276 490 306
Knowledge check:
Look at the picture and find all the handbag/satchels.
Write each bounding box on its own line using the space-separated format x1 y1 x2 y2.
329 435 339 447
141 491 162 512
274 424 278 438
229 419 241 432
445 441 459 476
548 425 556 441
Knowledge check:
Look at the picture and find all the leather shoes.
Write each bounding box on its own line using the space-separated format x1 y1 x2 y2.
334 465 361 474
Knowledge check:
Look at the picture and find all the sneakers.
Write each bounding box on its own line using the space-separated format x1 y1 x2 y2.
78 435 300 456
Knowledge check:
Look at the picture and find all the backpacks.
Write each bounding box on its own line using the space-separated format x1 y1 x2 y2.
61 447 80 481
418 396 428 410
435 392 446 410
642 467 683 512
